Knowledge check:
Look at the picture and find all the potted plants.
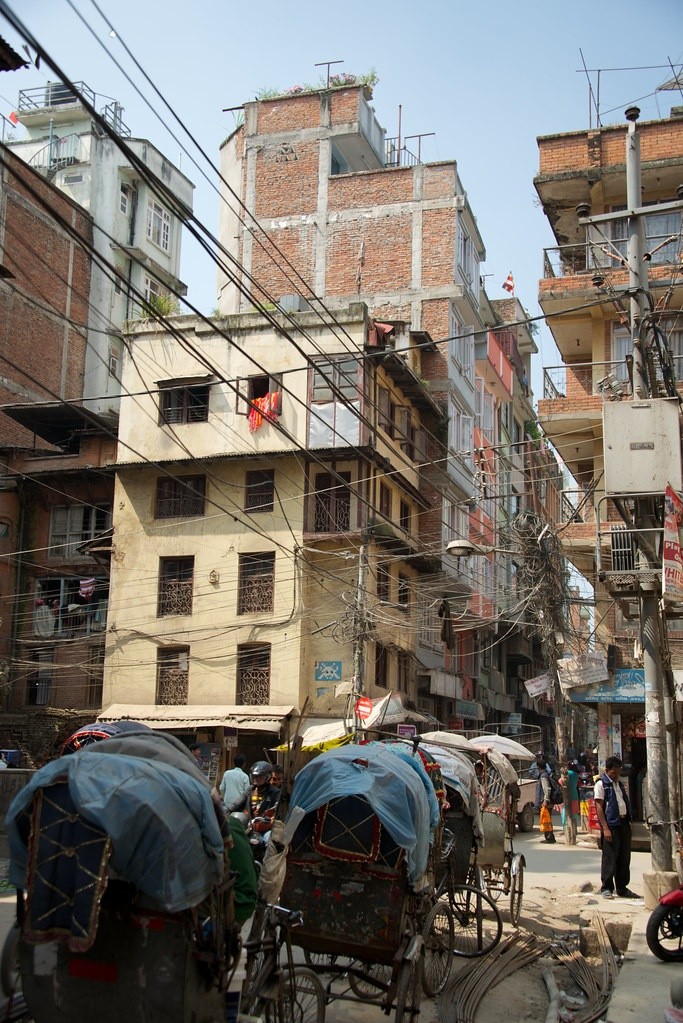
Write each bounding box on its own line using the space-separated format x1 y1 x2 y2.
359 63 379 94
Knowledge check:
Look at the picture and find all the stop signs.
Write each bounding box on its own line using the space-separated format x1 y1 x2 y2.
355 698 373 719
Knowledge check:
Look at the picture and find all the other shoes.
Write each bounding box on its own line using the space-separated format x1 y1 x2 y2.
618 888 640 898
540 839 556 843
539 805 553 832
602 890 614 899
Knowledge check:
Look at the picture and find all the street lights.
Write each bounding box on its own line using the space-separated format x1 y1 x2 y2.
444 540 576 847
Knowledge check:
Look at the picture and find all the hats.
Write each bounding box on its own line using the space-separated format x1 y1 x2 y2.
535 751 544 755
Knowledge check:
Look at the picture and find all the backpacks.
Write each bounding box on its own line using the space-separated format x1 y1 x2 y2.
548 777 563 804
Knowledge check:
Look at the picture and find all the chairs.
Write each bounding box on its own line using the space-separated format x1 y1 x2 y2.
477 762 510 821
441 784 475 876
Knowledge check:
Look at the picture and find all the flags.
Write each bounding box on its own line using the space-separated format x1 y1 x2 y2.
501 272 515 295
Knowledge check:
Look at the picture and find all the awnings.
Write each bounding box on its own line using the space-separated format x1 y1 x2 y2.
95 704 295 733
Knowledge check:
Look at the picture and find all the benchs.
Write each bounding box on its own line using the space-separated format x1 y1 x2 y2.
277 790 409 951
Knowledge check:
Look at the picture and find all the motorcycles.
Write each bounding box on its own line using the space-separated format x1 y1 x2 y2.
645 885 683 962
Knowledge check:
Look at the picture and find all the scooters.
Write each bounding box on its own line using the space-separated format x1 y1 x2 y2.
240 802 280 873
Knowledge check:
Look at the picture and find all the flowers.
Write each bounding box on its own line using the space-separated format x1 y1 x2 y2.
283 82 303 94
328 71 358 84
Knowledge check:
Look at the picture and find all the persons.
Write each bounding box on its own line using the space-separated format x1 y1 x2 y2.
530 743 598 844
190 743 203 767
218 755 291 845
593 757 640 900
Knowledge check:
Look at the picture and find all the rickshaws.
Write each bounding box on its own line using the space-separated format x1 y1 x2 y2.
1 722 542 1023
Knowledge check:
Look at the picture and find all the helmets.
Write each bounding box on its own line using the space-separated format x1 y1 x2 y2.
249 761 273 789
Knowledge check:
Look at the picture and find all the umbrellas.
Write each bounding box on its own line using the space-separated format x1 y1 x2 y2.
469 727 536 762
417 731 477 752
